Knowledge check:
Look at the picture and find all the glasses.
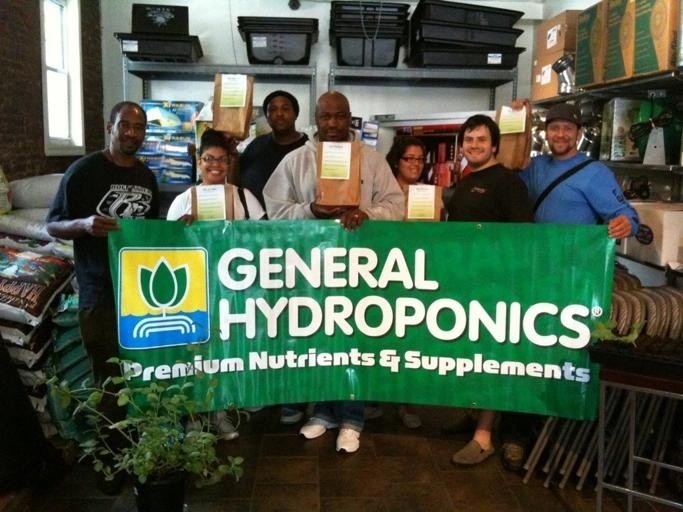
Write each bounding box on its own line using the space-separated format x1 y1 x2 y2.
200 153 230 164
401 155 425 163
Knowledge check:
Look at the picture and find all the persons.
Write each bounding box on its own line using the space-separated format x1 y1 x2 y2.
443 115 529 466
501 101 640 463
262 91 407 451
167 130 266 441
384 135 447 429
238 90 310 199
46 102 161 453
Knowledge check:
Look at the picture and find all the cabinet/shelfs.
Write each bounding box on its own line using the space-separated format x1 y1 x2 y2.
122 52 316 194
531 73 683 277
328 62 519 110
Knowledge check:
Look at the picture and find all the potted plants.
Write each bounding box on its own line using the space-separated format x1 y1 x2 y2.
47 356 250 512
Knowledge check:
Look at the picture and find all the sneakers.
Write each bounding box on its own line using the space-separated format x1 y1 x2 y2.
501 439 524 471
185 411 240 440
451 438 497 468
278 406 421 455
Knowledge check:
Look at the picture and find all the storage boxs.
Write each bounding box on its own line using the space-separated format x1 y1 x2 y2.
614 197 656 258
575 0 683 89
533 8 586 59
627 202 683 267
530 50 576 101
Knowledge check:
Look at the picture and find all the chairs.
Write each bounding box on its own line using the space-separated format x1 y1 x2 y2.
523 261 682 512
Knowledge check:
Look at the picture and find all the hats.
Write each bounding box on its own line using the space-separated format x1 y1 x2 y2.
545 105 581 127
